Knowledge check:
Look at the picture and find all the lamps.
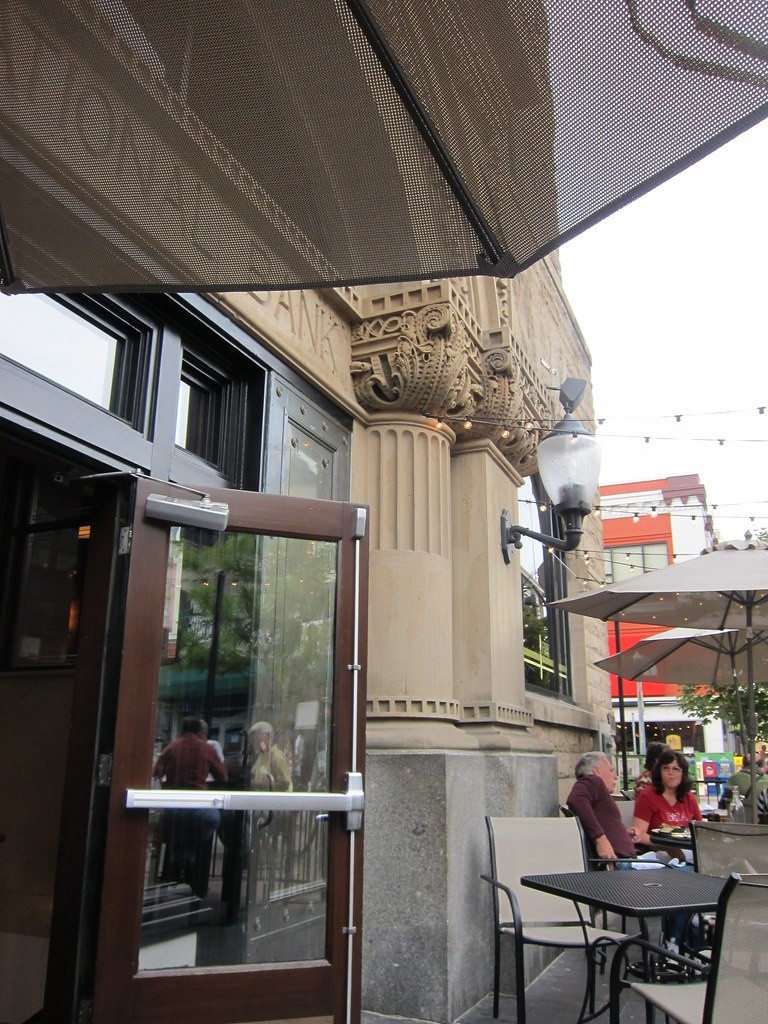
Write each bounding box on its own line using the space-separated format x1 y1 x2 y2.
502 377 602 563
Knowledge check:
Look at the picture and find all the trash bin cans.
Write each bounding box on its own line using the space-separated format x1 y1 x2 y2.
704 759 720 795
716 760 734 796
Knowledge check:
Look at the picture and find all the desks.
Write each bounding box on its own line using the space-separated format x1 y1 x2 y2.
519 868 728 1024
648 828 693 850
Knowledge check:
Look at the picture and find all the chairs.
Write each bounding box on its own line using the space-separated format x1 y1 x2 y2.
480 815 630 1024
561 790 768 1024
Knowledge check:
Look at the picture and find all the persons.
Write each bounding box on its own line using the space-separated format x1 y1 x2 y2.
718 746 768 815
632 750 704 864
633 741 672 799
148 716 295 896
566 750 701 947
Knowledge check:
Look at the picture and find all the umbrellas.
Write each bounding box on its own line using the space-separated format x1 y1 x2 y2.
593 627 768 758
158 660 267 700
0 1 767 300
544 538 768 824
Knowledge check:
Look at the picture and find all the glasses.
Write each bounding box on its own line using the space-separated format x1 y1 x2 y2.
659 764 682 773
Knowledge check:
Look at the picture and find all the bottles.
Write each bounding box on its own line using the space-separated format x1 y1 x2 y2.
728 785 746 824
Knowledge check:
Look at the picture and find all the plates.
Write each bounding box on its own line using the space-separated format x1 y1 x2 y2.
651 828 693 840
